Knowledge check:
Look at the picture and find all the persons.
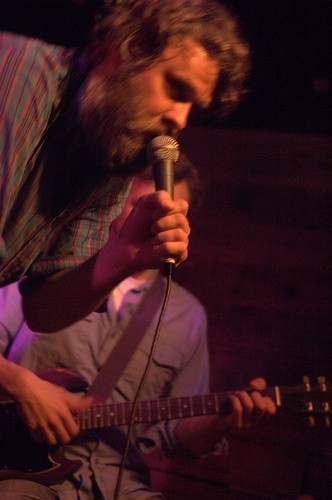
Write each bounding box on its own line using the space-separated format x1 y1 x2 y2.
1 152 276 500
1 0 252 335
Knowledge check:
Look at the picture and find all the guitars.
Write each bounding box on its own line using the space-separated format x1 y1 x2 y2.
1 365 332 486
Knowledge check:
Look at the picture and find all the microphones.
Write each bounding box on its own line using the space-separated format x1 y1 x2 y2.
150 135 179 275
